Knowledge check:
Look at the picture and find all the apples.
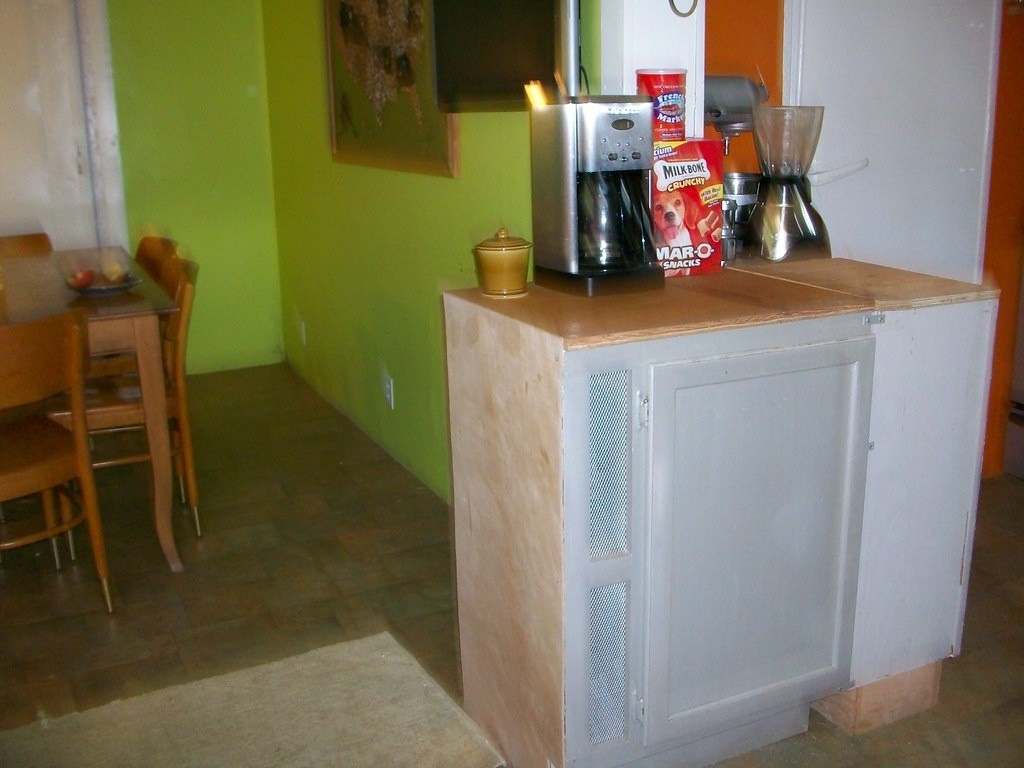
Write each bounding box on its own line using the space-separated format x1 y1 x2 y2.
65 270 93 289
101 264 129 285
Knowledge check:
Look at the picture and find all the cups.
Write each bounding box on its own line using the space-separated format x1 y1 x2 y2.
475 228 532 295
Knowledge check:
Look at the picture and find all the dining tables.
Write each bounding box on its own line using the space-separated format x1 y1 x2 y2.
0 245 186 573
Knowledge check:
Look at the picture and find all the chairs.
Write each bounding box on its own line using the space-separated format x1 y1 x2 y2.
0 231 54 263
135 236 179 282
1 316 116 616
44 253 200 561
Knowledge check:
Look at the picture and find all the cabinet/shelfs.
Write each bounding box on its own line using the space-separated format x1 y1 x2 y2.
441 256 1002 768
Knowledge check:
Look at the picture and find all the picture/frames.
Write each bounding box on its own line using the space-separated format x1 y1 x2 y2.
324 1 461 178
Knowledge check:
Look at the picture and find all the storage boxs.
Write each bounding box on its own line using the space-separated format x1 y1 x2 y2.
651 138 723 277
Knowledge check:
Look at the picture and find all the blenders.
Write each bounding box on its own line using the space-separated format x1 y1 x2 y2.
704 76 825 259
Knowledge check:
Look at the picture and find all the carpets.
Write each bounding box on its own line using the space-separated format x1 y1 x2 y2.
0 630 509 768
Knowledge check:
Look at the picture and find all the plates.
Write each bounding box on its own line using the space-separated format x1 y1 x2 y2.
64 273 142 294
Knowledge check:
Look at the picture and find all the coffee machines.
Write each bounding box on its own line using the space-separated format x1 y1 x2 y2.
529 95 664 280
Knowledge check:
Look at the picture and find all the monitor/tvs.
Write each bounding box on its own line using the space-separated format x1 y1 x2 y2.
433 0 582 113
